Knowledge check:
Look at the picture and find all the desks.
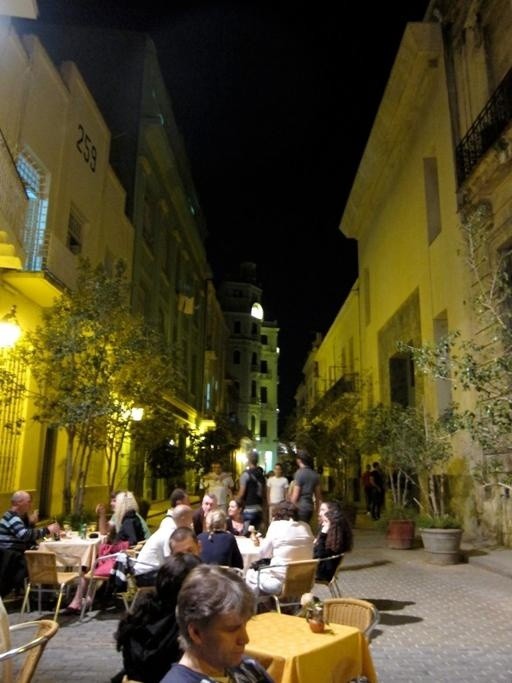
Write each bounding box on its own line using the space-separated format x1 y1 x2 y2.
204 532 268 570
237 610 378 682
26 528 108 616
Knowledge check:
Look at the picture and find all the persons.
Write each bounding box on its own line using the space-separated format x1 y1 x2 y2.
0 491 59 602
64 489 149 614
117 447 353 682
363 462 387 521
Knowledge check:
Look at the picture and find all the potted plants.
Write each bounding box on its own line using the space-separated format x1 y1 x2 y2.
383 489 416 550
417 513 464 565
331 486 358 529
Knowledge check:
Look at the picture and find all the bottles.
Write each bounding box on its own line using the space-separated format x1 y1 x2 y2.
53 520 60 541
307 603 325 634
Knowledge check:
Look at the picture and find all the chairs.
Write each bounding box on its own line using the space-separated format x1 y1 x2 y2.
297 597 379 639
2 619 57 681
79 549 135 620
138 539 147 544
253 559 319 614
316 554 345 598
22 550 81 622
130 544 144 552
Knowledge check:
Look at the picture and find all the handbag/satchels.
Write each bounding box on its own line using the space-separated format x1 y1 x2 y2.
96 541 130 577
